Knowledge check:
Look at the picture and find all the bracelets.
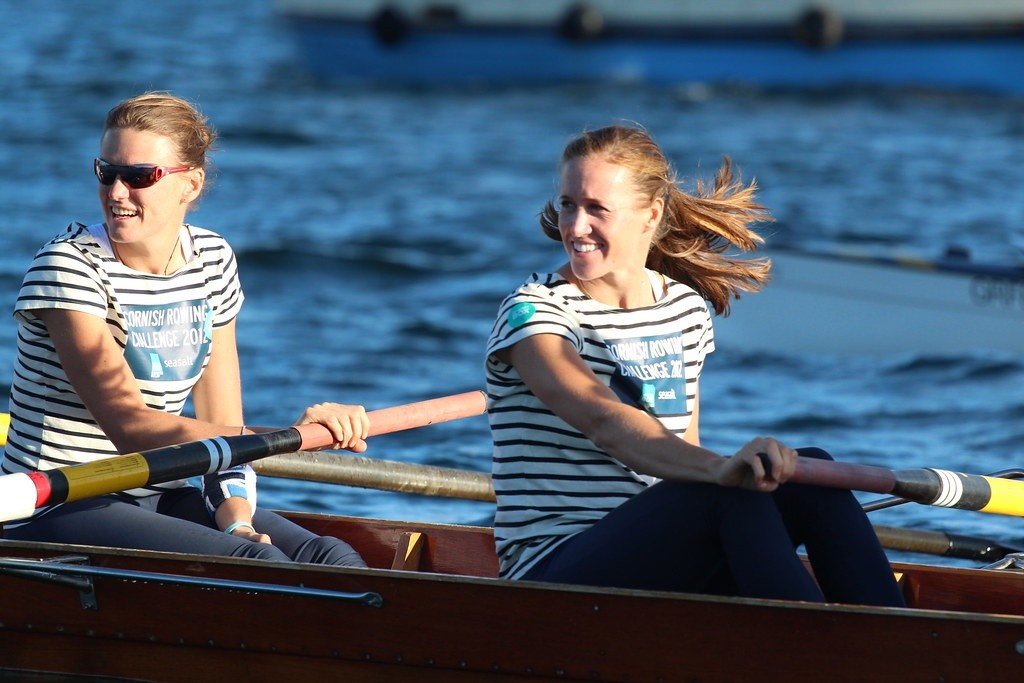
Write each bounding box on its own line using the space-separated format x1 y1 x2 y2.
239 426 246 435
223 521 255 537
0 91 372 576
485 122 906 614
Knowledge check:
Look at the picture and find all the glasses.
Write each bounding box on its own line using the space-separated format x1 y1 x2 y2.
94 156 204 189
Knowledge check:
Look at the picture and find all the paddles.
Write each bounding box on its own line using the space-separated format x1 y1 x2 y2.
754 449 1024 517
0 390 487 519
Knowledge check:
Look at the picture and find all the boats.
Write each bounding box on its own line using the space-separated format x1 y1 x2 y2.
0 510 1024 683
